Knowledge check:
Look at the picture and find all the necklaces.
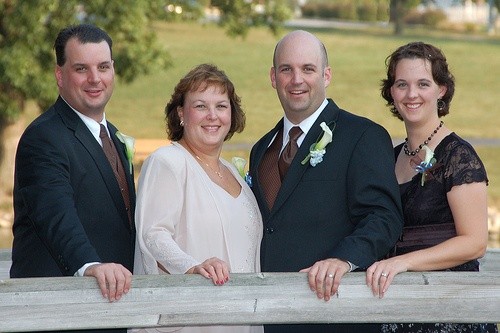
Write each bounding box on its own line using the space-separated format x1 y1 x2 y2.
404 120 444 155
186 142 224 179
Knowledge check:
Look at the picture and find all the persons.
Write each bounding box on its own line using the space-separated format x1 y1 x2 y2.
127 64 265 333
9 25 136 333
366 42 498 333
249 30 405 333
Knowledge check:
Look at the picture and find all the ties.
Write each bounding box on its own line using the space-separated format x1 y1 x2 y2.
99 123 118 172
278 126 304 183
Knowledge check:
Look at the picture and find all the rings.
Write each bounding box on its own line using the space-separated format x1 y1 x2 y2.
382 274 388 277
327 274 334 279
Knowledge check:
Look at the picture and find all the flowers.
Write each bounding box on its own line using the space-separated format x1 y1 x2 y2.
232 156 253 185
413 146 439 186
298 123 332 168
115 130 137 171
